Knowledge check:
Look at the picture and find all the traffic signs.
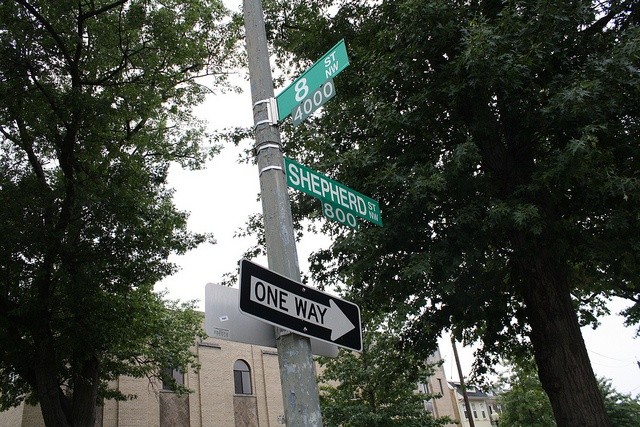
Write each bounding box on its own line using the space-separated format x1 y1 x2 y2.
239 258 363 351
276 38 350 128
284 156 384 231
